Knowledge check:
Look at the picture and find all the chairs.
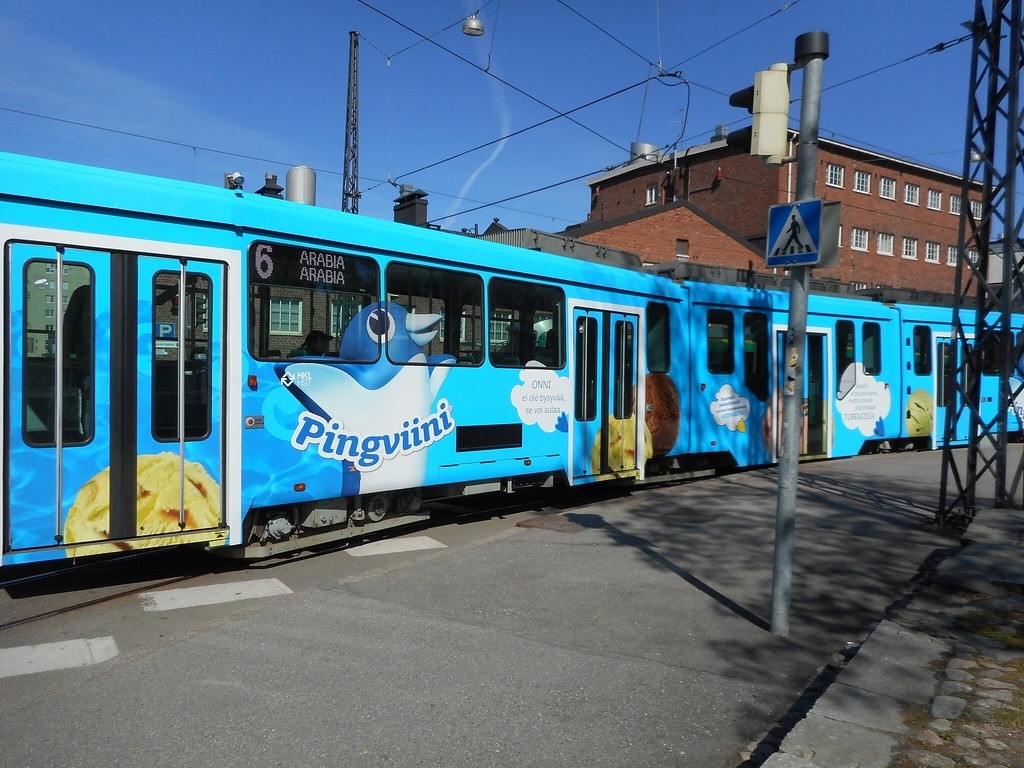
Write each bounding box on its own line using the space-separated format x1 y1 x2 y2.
265 350 564 368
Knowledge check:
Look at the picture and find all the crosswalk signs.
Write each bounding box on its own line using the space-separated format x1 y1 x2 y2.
765 197 822 269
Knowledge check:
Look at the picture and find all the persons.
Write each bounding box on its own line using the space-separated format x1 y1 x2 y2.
302 330 331 355
62 283 94 439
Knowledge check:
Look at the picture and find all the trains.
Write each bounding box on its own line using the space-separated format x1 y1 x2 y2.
1 150 1023 569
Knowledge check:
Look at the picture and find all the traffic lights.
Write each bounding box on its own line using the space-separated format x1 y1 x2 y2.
724 68 790 160
